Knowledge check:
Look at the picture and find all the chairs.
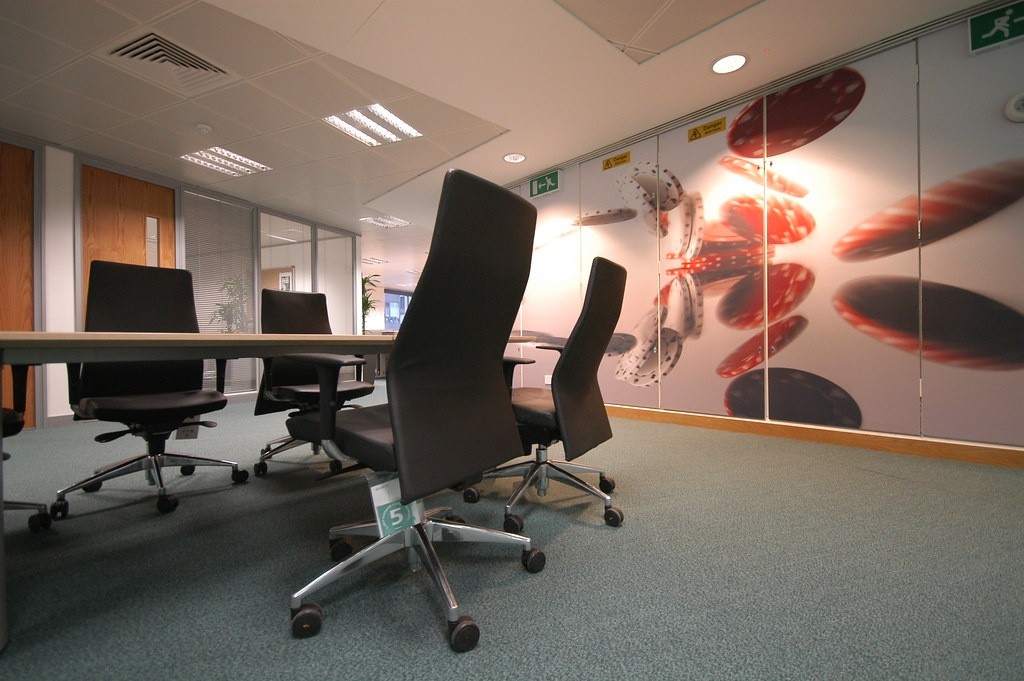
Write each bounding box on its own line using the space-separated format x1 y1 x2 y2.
254 289 375 481
286 166 546 652
51 259 250 520
0 362 50 532
463 258 625 534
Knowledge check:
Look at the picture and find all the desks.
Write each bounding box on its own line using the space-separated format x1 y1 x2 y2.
0 330 535 364
